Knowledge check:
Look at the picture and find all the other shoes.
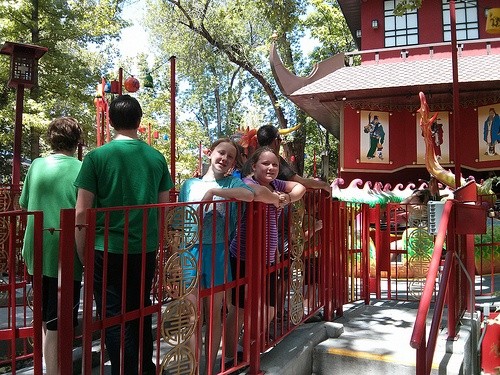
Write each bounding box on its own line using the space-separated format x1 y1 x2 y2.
225 358 246 375
271 309 288 322
304 311 323 323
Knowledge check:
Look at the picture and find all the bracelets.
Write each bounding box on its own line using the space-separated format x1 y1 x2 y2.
288 193 291 204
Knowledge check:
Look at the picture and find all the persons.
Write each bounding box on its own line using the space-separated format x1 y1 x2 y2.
241 125 330 320
223 146 306 375
74 94 174 375
431 116 443 161
304 191 323 322
483 108 500 157
18 118 82 375
171 137 254 375
362 113 386 160
